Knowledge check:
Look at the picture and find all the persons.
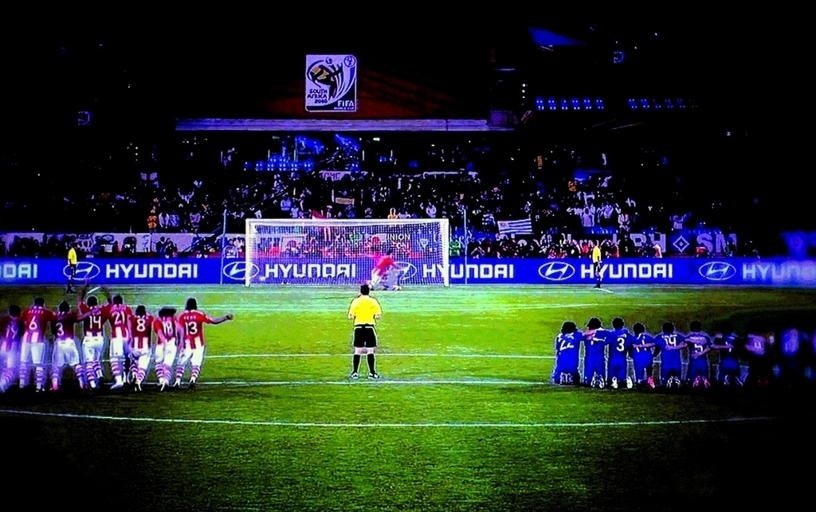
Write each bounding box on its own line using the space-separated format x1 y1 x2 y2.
347 284 382 380
551 316 816 395
366 249 403 291
0 282 236 393
63 243 80 295
364 246 389 291
1 111 812 260
383 265 404 291
591 241 603 288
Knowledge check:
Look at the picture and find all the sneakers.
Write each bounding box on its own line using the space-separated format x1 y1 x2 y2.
349 374 358 379
2 379 197 394
367 373 381 379
558 372 744 391
